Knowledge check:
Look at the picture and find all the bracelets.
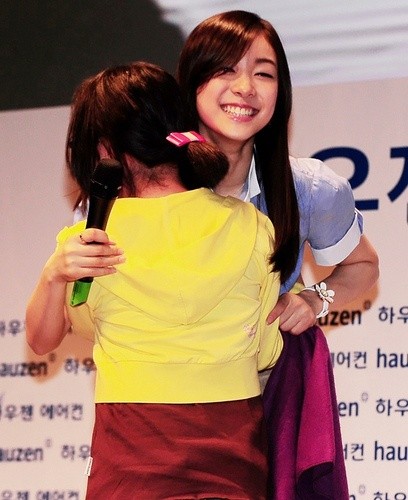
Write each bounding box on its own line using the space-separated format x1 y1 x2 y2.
301 281 334 319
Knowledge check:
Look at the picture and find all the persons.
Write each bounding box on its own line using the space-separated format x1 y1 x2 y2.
25 11 378 500
56 60 304 500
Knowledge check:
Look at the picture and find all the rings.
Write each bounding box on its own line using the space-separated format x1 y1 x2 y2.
79 231 85 242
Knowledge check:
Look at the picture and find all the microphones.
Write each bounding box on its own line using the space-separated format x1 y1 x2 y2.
70 157 123 307
